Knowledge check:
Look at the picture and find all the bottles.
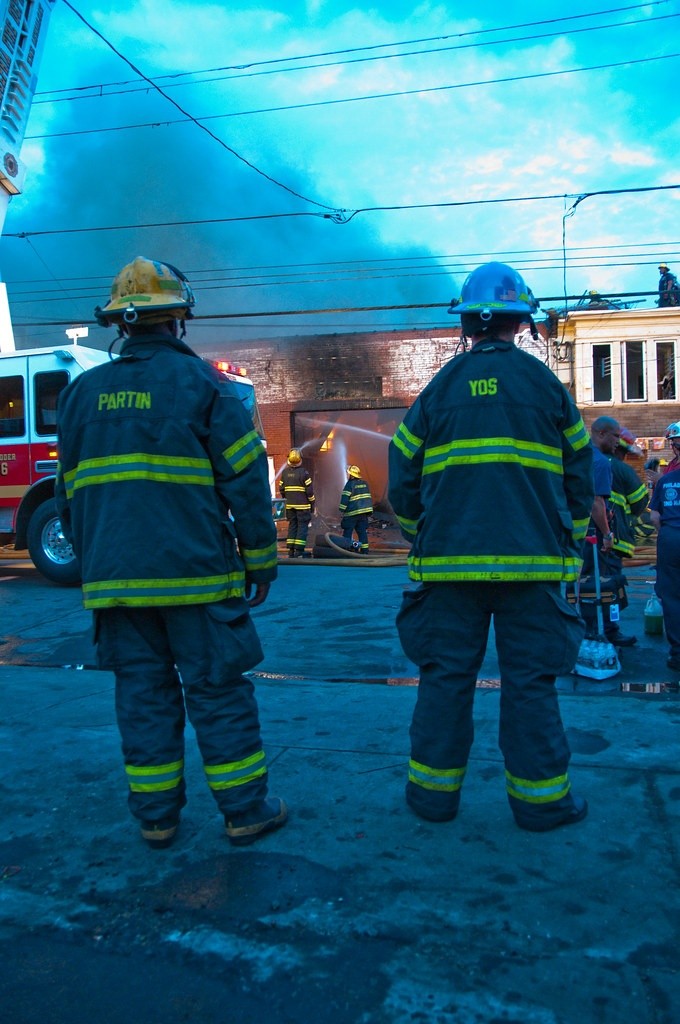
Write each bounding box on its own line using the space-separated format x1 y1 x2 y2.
644 593 663 635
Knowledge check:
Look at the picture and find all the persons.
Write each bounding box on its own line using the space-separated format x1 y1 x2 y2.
581 415 650 645
55 254 287 847
657 263 680 307
643 420 680 669
338 465 373 555
278 449 316 558
388 261 593 832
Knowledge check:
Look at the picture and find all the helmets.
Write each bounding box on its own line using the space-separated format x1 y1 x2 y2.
617 427 634 451
287 450 302 468
346 465 362 479
101 256 196 320
447 261 538 315
666 420 680 439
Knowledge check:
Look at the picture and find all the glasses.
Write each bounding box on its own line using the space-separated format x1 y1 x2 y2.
671 442 680 450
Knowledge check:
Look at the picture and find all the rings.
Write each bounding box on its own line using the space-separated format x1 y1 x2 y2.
651 476 653 477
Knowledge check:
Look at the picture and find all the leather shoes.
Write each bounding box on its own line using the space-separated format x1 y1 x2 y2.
558 796 588 827
610 629 637 647
666 656 675 669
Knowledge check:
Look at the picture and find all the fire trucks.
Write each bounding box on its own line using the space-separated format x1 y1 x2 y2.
0 0 288 589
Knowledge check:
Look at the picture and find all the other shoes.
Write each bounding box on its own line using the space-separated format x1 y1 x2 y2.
140 812 180 849
288 549 311 558
224 796 286 848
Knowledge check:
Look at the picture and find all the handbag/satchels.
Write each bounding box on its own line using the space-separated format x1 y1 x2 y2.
567 575 629 623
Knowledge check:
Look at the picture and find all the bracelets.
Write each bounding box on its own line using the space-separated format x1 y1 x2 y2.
603 532 612 540
